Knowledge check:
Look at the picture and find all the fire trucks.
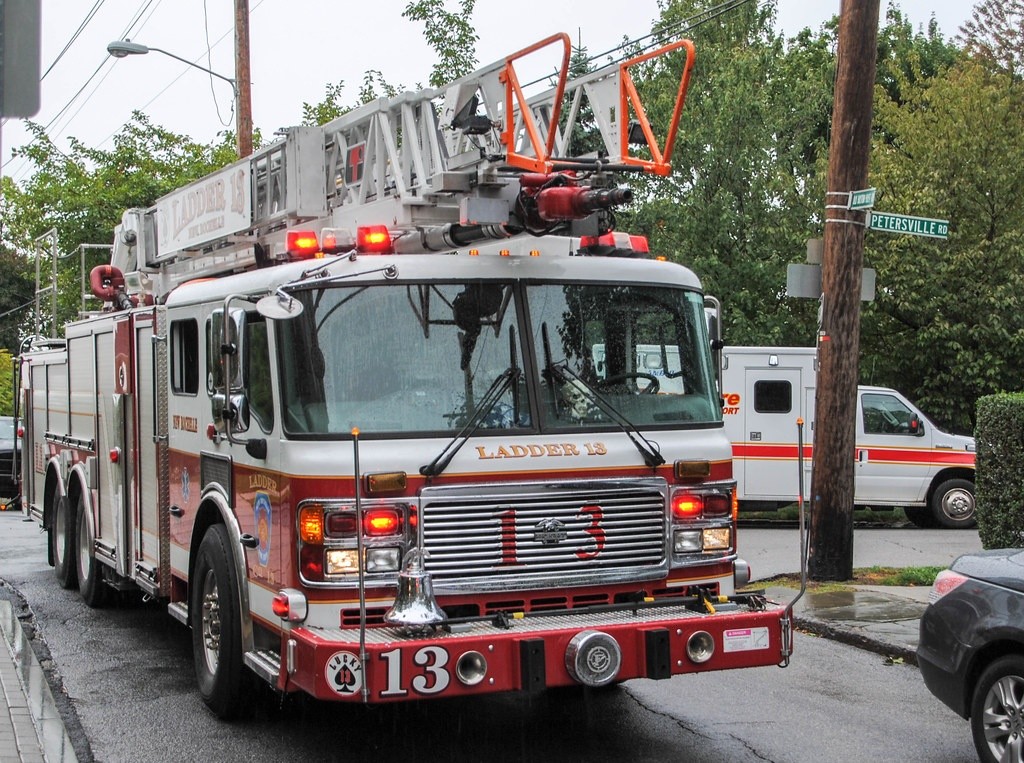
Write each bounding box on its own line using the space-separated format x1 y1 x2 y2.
13 32 815 725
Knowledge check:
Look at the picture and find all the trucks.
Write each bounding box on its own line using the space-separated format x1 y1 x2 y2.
591 338 979 530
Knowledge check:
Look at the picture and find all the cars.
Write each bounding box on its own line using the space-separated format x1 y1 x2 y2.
0 414 25 498
916 544 1024 763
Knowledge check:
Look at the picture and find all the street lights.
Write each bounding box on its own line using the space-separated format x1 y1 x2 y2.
107 36 256 159
